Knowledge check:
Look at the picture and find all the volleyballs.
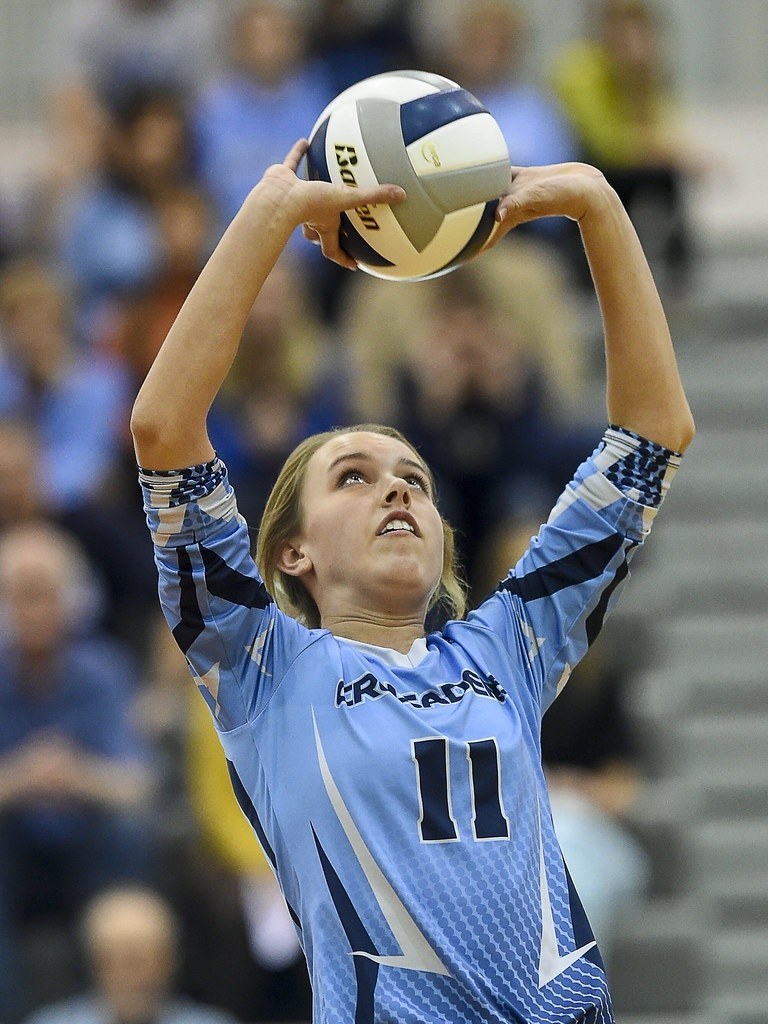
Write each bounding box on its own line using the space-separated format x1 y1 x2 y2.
303 69 510 284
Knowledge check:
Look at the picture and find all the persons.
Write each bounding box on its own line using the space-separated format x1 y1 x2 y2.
27 883 233 1024
0 0 704 1024
130 139 696 1024
